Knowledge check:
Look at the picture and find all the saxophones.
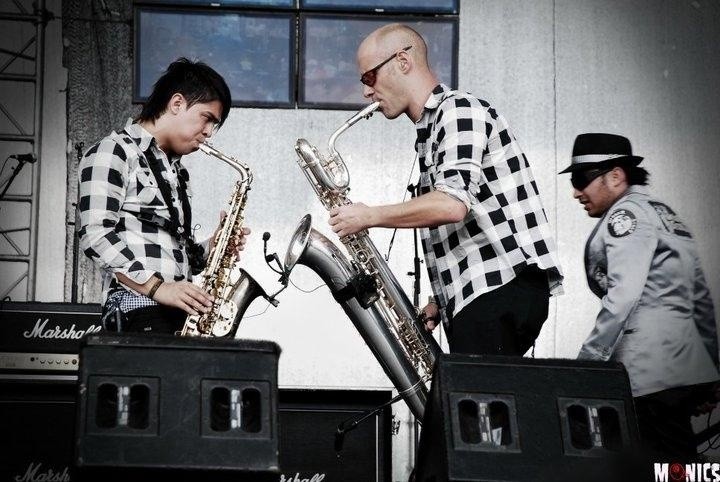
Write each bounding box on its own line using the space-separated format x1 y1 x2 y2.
180 140 269 340
285 100 445 424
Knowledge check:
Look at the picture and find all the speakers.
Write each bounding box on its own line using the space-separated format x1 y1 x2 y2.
0 374 79 482
71 335 282 482
259 406 384 482
417 353 643 482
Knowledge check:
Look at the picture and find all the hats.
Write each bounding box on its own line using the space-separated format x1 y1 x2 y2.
558 132 643 176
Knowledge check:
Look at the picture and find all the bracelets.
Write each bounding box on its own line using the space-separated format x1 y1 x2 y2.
146 277 164 299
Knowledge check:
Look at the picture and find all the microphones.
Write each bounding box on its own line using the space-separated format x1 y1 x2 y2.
269 285 289 300
337 422 356 435
263 232 286 281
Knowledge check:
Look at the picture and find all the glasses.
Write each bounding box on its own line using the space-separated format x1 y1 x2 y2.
360 44 413 88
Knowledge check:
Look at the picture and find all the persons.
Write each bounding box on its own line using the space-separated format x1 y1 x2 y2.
327 15 567 481
556 132 720 482
76 54 252 347
